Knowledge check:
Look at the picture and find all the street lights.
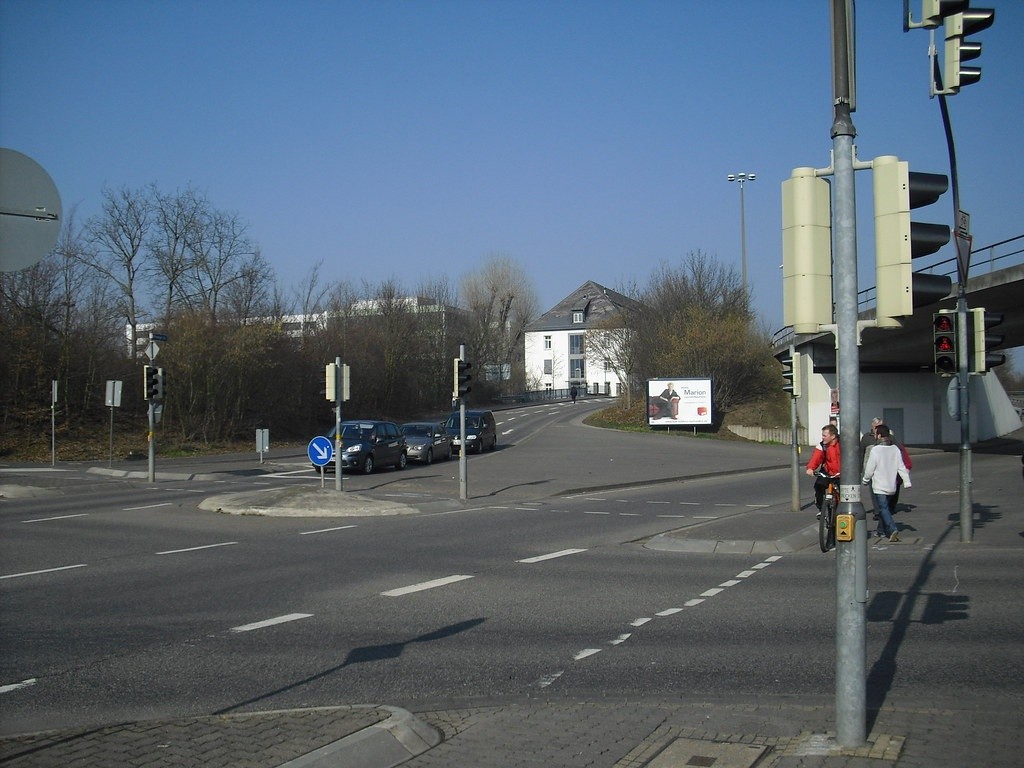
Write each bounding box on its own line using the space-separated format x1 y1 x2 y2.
726 172 758 314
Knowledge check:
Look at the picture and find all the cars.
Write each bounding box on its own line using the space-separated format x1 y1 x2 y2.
398 421 454 466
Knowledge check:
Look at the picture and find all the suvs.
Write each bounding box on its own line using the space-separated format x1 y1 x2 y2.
311 419 408 475
441 409 497 455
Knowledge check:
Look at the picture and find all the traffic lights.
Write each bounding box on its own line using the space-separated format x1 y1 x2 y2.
943 6 996 41
932 310 959 374
921 1 970 30
869 155 952 329
319 362 337 403
942 35 984 91
973 305 1008 374
781 351 802 398
142 364 158 401
452 357 473 398
153 367 167 400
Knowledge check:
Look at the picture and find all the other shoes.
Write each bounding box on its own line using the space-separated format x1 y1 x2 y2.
874 533 884 538
816 511 821 517
889 529 899 541
872 513 879 520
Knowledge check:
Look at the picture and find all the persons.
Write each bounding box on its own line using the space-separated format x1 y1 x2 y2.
472 418 477 427
805 423 841 516
861 424 912 542
860 417 912 520
830 417 837 428
570 387 577 403
653 382 681 420
831 390 840 415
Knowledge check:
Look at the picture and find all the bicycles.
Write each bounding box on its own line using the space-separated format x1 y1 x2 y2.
810 470 841 553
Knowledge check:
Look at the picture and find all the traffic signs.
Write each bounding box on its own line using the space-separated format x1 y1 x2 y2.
153 333 168 342
